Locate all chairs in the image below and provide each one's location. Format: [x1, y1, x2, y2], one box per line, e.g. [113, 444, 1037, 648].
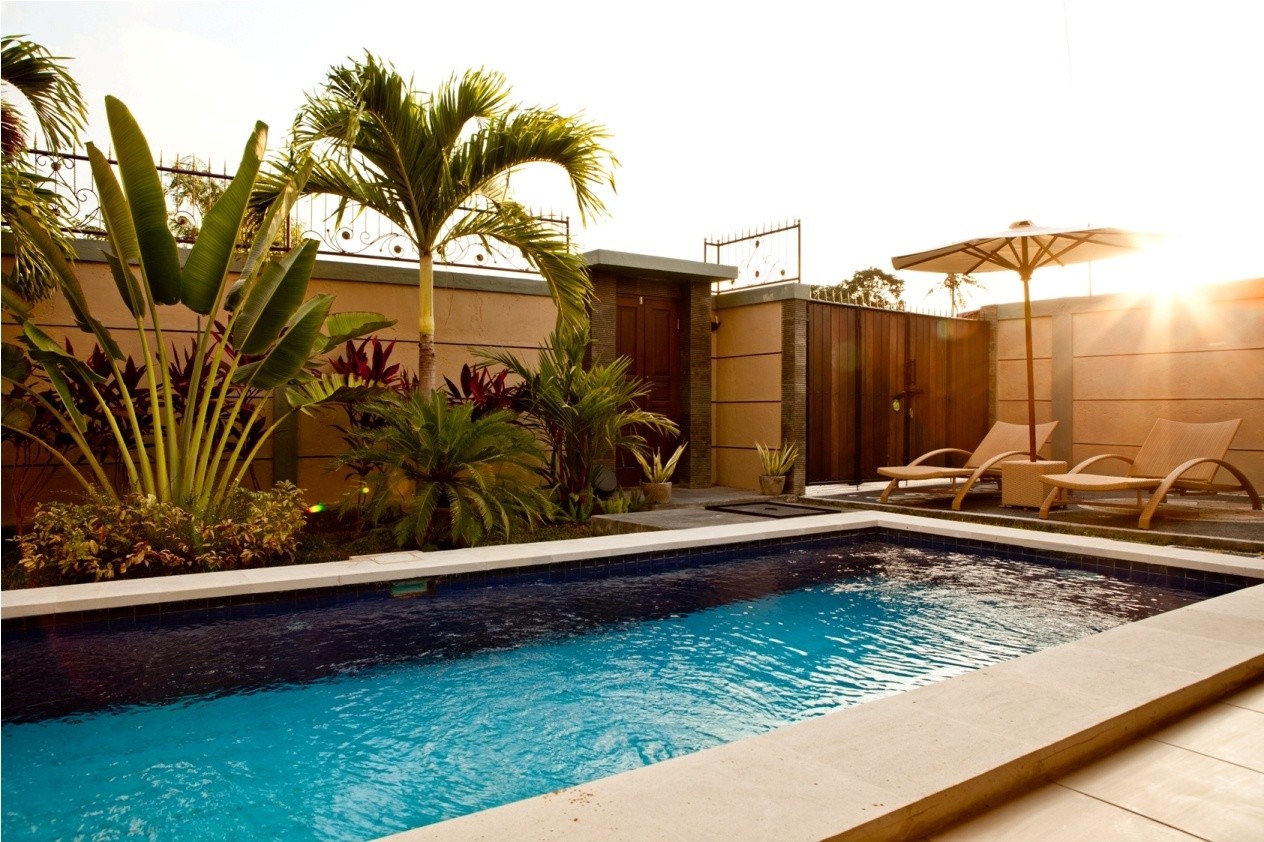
[1038, 418, 1263, 529]
[877, 420, 1059, 511]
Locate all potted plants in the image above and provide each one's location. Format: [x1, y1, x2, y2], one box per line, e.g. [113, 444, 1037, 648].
[629, 440, 689, 504]
[754, 438, 801, 495]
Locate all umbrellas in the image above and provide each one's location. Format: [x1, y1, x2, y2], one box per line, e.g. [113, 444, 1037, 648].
[891, 219, 1156, 462]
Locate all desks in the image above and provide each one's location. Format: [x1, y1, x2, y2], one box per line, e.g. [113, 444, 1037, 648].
[1001, 460, 1068, 510]
